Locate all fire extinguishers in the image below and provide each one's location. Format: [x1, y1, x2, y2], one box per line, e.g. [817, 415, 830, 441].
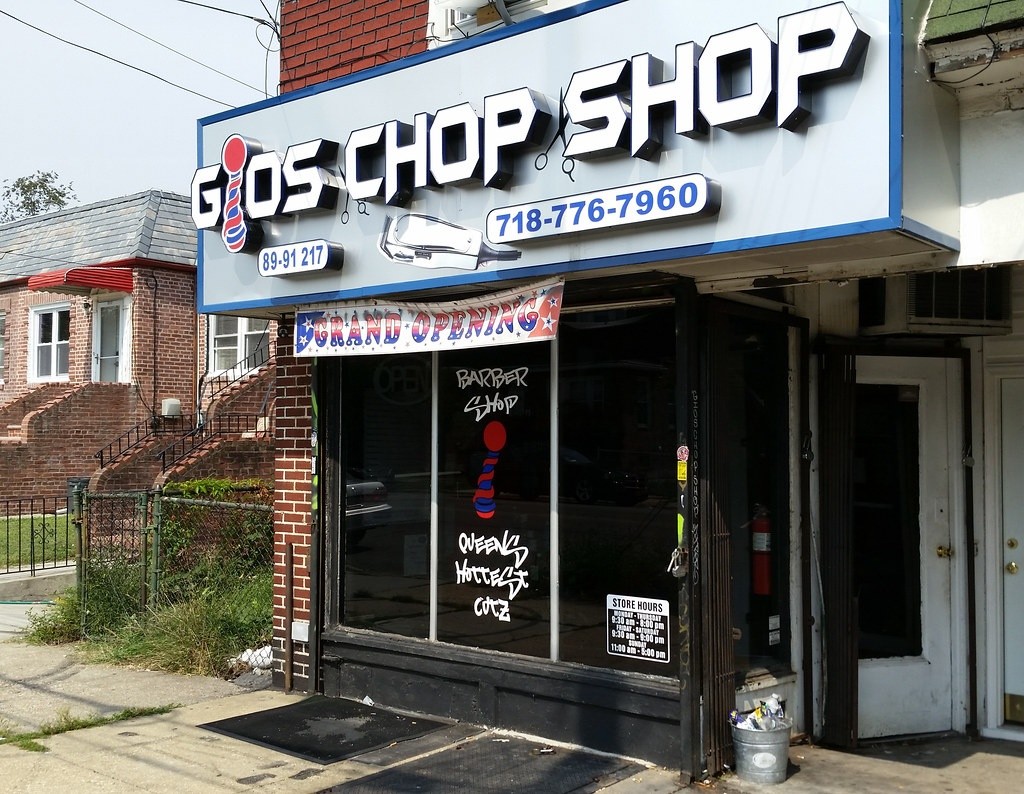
[750, 500, 775, 597]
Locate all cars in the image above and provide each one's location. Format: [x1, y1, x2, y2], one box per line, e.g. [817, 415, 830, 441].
[343, 470, 392, 551]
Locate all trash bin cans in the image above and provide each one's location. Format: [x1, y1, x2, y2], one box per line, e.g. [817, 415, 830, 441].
[728, 709, 795, 787]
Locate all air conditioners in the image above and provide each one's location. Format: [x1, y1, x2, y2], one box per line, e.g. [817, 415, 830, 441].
[859, 264, 1014, 337]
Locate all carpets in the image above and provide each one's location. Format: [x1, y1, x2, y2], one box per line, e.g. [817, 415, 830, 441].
[195, 695, 454, 766]
